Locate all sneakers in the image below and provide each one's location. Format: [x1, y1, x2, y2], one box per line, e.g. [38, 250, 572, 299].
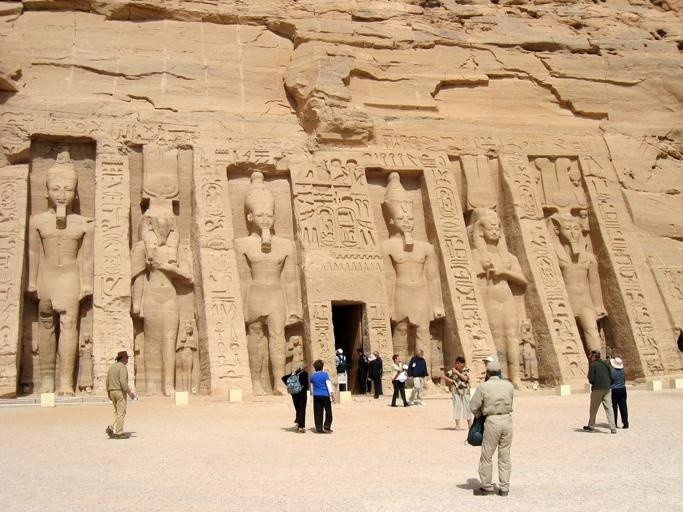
[111, 435, 127, 439]
[498, 489, 508, 496]
[105, 425, 113, 438]
[390, 402, 423, 407]
[473, 487, 494, 495]
[610, 424, 628, 434]
[294, 424, 333, 434]
[582, 426, 594, 432]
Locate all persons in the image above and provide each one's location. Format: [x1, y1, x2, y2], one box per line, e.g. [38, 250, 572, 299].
[609, 357, 628, 428]
[379, 171, 446, 373]
[548, 212, 609, 359]
[233, 170, 305, 396]
[440, 357, 472, 431]
[79, 332, 93, 392]
[368, 351, 384, 399]
[281, 364, 309, 433]
[407, 350, 429, 405]
[389, 354, 409, 407]
[104, 351, 135, 439]
[520, 319, 539, 380]
[176, 320, 196, 392]
[336, 348, 347, 373]
[482, 357, 504, 382]
[468, 361, 515, 497]
[24, 144, 93, 398]
[356, 349, 368, 395]
[466, 208, 527, 389]
[286, 336, 303, 373]
[130, 214, 193, 397]
[309, 359, 336, 434]
[583, 350, 617, 433]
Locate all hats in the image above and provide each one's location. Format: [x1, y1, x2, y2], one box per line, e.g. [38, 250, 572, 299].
[609, 358, 624, 370]
[115, 350, 130, 359]
[588, 351, 600, 359]
[482, 357, 495, 361]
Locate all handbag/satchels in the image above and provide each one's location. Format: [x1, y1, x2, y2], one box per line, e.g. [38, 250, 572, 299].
[466, 415, 486, 446]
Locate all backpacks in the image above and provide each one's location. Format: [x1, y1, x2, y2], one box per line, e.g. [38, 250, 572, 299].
[336, 355, 347, 371]
[286, 370, 304, 394]
